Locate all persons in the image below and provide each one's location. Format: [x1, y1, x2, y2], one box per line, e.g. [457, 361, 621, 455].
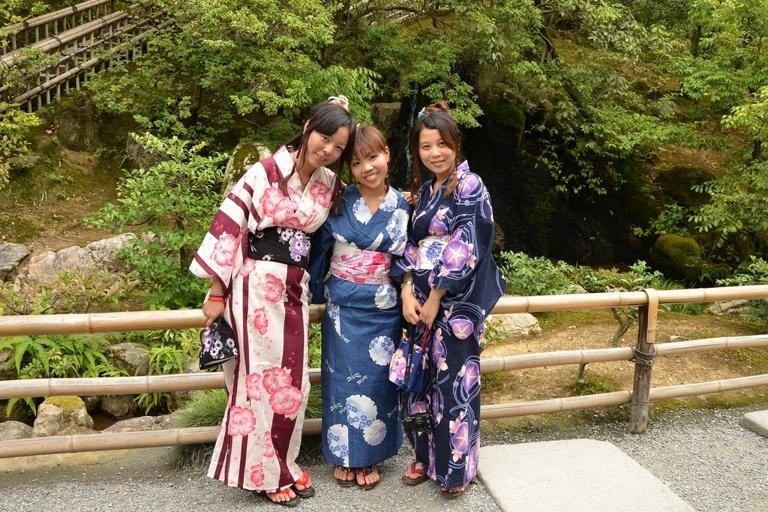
[389, 102, 507, 498]
[309, 124, 413, 490]
[189, 94, 419, 507]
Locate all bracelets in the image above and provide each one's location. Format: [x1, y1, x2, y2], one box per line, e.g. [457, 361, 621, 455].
[401, 279, 414, 285]
[207, 295, 225, 302]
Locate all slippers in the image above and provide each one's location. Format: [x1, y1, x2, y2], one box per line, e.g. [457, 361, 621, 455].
[402, 460, 430, 485]
[439, 479, 472, 498]
[265, 470, 315, 507]
[333, 465, 381, 491]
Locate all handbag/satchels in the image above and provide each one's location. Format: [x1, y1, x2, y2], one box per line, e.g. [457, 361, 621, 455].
[388, 320, 429, 396]
[199, 316, 239, 370]
